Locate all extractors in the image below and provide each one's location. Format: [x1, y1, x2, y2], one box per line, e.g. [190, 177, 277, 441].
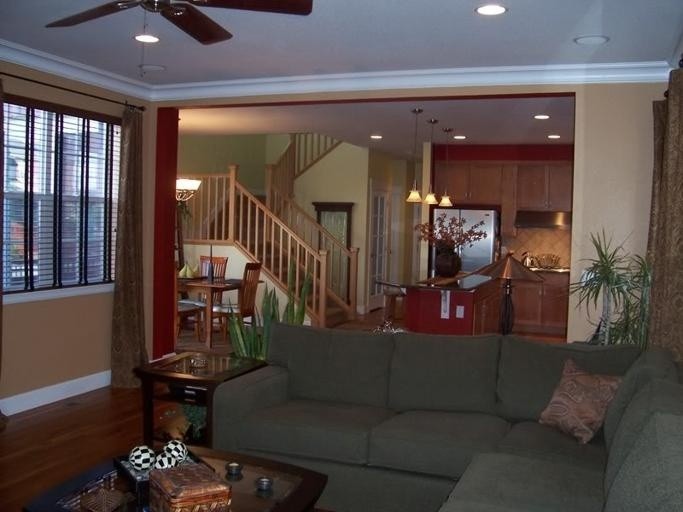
[514, 210, 572, 230]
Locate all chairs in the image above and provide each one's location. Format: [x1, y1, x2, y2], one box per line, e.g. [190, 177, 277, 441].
[175, 255, 263, 348]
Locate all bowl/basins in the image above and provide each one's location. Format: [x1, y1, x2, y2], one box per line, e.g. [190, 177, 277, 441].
[535, 254, 560, 269]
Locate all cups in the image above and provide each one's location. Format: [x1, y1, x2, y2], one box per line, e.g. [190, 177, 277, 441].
[255, 476, 273, 491]
[224, 461, 242, 475]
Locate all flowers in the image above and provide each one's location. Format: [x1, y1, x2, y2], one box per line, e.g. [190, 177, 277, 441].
[412, 213, 488, 253]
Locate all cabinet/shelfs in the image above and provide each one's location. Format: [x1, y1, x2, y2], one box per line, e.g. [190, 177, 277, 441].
[517, 164, 572, 208]
[435, 162, 501, 202]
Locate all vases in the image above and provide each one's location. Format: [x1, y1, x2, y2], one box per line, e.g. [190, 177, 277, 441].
[434, 247, 462, 278]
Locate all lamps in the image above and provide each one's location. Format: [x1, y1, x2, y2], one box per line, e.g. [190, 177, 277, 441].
[477, 252, 543, 336]
[405, 104, 453, 207]
[175, 178, 201, 202]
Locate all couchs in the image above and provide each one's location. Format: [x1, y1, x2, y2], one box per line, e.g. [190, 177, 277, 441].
[208, 320, 683, 511]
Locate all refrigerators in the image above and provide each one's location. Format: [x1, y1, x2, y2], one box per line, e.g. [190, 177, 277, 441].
[431, 209, 500, 275]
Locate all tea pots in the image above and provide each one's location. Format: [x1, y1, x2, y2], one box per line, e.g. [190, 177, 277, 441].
[521, 251, 535, 268]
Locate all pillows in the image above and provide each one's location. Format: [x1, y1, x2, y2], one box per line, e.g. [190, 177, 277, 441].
[539, 358, 624, 446]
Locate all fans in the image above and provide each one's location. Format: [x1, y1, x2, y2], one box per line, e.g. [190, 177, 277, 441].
[39, 0, 317, 45]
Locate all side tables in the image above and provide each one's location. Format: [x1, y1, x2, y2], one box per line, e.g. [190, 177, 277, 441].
[131, 349, 267, 451]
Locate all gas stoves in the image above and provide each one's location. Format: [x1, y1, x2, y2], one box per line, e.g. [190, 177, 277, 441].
[526, 267, 570, 273]
